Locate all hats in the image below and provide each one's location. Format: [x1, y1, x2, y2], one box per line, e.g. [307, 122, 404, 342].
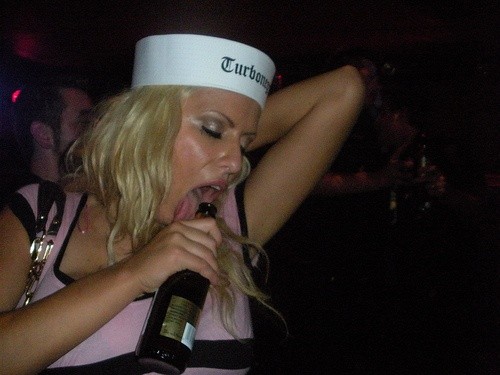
[133, 31, 275, 109]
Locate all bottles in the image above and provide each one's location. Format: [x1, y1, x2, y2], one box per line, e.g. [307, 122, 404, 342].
[415, 142, 429, 168]
[135, 202, 219, 374]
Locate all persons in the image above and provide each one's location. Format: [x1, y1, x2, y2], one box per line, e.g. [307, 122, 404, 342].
[1, 30, 368, 375]
[250, 43, 444, 375]
[0, 69, 94, 202]
